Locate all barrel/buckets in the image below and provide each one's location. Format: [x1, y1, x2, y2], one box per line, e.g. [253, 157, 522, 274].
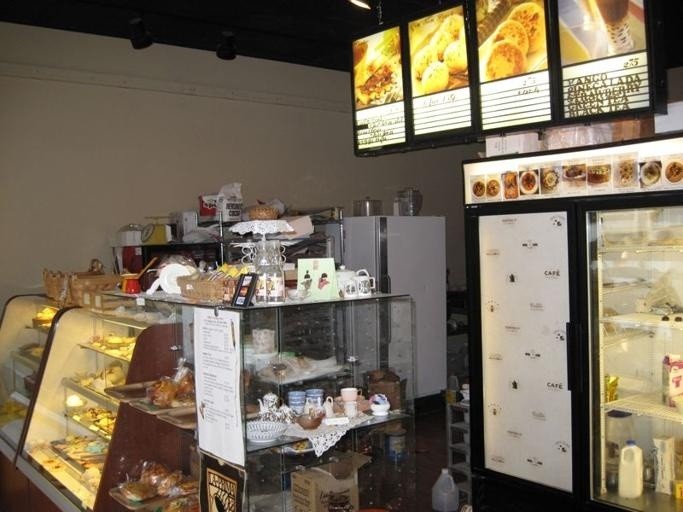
[618, 440, 644, 497]
[432, 468, 458, 511]
[398, 190, 421, 216]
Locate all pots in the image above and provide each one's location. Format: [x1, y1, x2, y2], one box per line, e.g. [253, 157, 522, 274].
[352, 196, 383, 216]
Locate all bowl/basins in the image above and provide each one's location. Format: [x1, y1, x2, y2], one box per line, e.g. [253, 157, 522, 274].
[485, 180, 499, 198]
[639, 162, 660, 186]
[663, 160, 682, 183]
[286, 389, 324, 415]
[471, 182, 485, 199]
[245, 419, 288, 444]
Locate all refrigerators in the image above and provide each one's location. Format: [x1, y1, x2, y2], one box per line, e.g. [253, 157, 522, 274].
[460, 129, 679, 512]
[324, 216, 447, 416]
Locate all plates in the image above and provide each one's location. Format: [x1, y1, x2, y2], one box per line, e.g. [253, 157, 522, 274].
[561, 171, 586, 180]
[333, 395, 365, 405]
[519, 170, 539, 196]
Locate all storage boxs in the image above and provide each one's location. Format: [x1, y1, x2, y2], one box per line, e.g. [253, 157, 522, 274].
[363, 369, 407, 412]
[289, 452, 370, 511]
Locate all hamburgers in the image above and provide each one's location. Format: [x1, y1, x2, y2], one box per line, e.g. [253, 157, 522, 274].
[354, 52, 397, 105]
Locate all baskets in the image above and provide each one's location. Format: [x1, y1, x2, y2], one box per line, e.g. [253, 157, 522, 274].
[70, 271, 126, 307]
[176, 275, 233, 303]
[41, 267, 76, 307]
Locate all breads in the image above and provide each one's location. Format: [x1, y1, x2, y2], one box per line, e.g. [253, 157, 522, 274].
[148, 372, 195, 407]
[413, 14, 468, 94]
[119, 464, 200, 512]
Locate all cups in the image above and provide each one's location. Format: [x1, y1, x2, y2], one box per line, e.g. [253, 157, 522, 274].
[340, 387, 363, 400]
[370, 403, 390, 416]
[296, 413, 321, 430]
[336, 265, 377, 298]
[119, 273, 141, 293]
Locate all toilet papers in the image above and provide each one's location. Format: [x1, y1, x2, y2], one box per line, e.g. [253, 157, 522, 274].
[146, 264, 189, 296]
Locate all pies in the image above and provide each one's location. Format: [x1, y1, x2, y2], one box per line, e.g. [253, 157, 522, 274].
[486, 2, 546, 81]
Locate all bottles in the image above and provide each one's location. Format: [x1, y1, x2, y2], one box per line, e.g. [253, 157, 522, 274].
[253, 255, 285, 302]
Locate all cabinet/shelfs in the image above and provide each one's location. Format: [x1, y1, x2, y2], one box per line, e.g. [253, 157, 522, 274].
[444, 402, 471, 505]
[191, 289, 417, 512]
[1, 292, 68, 511]
[7, 307, 192, 511]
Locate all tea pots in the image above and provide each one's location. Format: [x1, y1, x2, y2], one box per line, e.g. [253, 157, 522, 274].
[256, 392, 283, 418]
